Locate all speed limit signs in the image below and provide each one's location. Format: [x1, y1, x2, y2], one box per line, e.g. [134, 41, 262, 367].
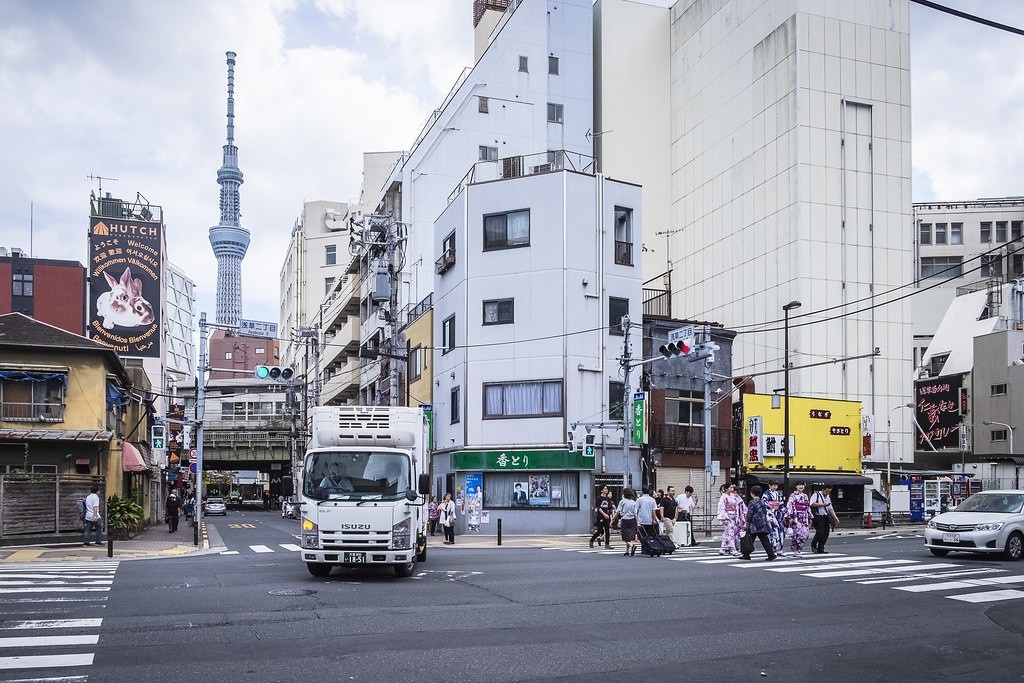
[189, 448, 197, 459]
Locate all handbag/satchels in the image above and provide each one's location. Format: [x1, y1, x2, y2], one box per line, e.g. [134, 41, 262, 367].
[828, 513, 834, 523]
[738, 530, 754, 553]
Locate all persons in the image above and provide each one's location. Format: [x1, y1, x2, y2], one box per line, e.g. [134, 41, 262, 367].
[83, 486, 106, 547]
[676, 485, 700, 546]
[615, 485, 664, 557]
[438, 493, 456, 544]
[597, 491, 617, 546]
[165, 489, 182, 533]
[429, 496, 440, 536]
[717, 482, 839, 562]
[183, 493, 194, 515]
[319, 462, 354, 491]
[589, 485, 615, 549]
[238, 493, 244, 501]
[530, 476, 549, 497]
[475, 485, 481, 498]
[370, 462, 401, 492]
[513, 483, 526, 501]
[263, 492, 284, 510]
[660, 486, 679, 549]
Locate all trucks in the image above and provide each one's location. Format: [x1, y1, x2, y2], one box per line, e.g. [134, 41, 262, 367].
[282, 405, 431, 577]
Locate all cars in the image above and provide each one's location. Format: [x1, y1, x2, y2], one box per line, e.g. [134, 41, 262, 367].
[227, 492, 240, 503]
[203, 498, 227, 516]
[923, 490, 1024, 561]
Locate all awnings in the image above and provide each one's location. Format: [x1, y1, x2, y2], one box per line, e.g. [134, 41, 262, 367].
[122, 442, 153, 475]
[748, 474, 873, 485]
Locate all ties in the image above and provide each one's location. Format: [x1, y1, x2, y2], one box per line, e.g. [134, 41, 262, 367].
[445, 504, 448, 520]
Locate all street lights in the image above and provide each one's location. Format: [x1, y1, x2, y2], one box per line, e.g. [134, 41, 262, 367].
[887, 402, 917, 493]
[983, 420, 1013, 454]
[783, 300, 802, 508]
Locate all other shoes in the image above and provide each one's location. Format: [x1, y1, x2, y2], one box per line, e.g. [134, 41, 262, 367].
[778, 552, 787, 557]
[719, 550, 724, 555]
[589, 540, 594, 548]
[444, 542, 454, 544]
[631, 546, 637, 556]
[693, 542, 699, 546]
[83, 543, 93, 547]
[605, 544, 614, 549]
[818, 550, 828, 554]
[811, 546, 818, 554]
[765, 555, 776, 561]
[739, 555, 750, 560]
[624, 553, 629, 556]
[96, 542, 105, 547]
[733, 552, 739, 556]
[794, 551, 801, 556]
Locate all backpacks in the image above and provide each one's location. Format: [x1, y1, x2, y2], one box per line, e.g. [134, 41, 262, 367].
[78, 498, 87, 519]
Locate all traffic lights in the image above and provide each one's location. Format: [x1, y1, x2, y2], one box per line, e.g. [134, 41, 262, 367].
[582, 434, 596, 457]
[567, 431, 578, 453]
[255, 365, 295, 382]
[659, 338, 693, 360]
[151, 425, 166, 451]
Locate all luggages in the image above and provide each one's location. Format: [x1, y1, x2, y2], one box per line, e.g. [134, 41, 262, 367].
[637, 525, 663, 557]
[675, 519, 692, 547]
[652, 524, 676, 554]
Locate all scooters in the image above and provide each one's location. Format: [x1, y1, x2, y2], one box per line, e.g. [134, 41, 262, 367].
[281, 500, 295, 519]
